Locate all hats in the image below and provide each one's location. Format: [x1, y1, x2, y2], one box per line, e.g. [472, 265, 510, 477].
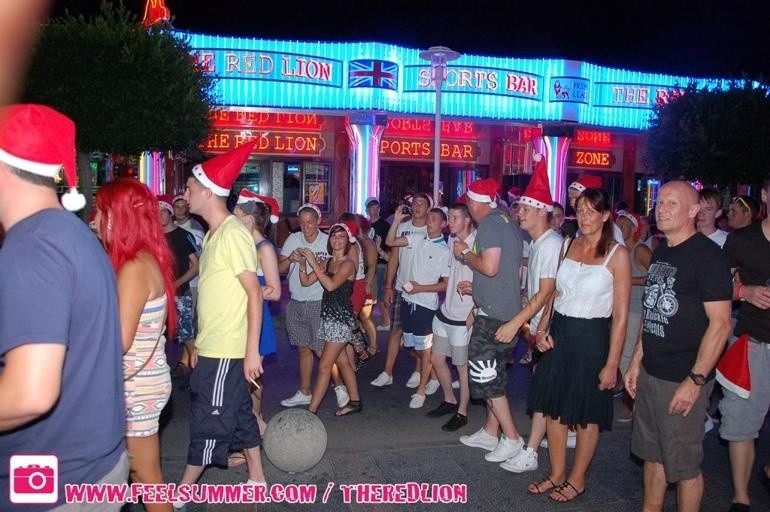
[172, 192, 185, 205]
[508, 185, 522, 198]
[157, 193, 175, 217]
[236, 188, 280, 225]
[519, 152, 554, 213]
[1, 102, 87, 212]
[297, 201, 322, 219]
[328, 220, 357, 243]
[616, 209, 643, 240]
[467, 177, 500, 208]
[568, 174, 602, 193]
[192, 136, 260, 198]
[715, 332, 752, 400]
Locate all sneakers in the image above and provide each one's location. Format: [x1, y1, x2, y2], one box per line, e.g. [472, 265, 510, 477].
[408, 394, 426, 409]
[459, 426, 499, 452]
[424, 379, 441, 396]
[425, 401, 459, 418]
[405, 371, 421, 389]
[485, 432, 526, 463]
[281, 389, 313, 408]
[441, 413, 468, 432]
[370, 371, 394, 387]
[617, 407, 633, 423]
[376, 324, 391, 332]
[539, 428, 577, 449]
[498, 444, 539, 474]
[333, 385, 350, 408]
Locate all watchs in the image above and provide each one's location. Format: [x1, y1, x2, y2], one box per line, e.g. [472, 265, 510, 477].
[688, 370, 706, 386]
[460, 249, 472, 263]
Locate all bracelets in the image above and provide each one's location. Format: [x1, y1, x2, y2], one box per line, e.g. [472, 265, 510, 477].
[300, 270, 307, 273]
[536, 330, 546, 333]
[316, 270, 323, 277]
[736, 284, 744, 300]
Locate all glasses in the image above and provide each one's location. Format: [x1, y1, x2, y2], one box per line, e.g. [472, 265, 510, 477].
[576, 208, 596, 216]
[733, 196, 751, 212]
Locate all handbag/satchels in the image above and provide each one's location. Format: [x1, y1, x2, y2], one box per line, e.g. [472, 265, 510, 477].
[325, 257, 367, 354]
[530, 235, 576, 364]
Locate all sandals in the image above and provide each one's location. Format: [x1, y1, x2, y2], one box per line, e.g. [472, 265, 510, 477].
[366, 345, 381, 356]
[334, 398, 364, 417]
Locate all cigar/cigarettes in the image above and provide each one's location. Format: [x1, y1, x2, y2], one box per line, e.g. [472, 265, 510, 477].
[249, 377, 261, 388]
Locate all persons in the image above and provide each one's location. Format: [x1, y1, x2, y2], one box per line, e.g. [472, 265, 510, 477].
[366, 197, 393, 331]
[724, 195, 759, 228]
[385, 202, 441, 410]
[428, 203, 478, 430]
[354, 212, 381, 358]
[173, 140, 267, 507]
[494, 154, 565, 473]
[233, 189, 283, 436]
[297, 218, 363, 417]
[623, 180, 733, 512]
[93, 180, 178, 511]
[527, 185, 632, 502]
[453, 178, 525, 462]
[338, 220, 367, 372]
[618, 214, 652, 422]
[570, 176, 626, 248]
[154, 195, 199, 374]
[371, 195, 434, 387]
[696, 189, 729, 246]
[720, 172, 770, 512]
[1, 105, 131, 512]
[173, 198, 205, 313]
[278, 202, 350, 409]
[551, 201, 564, 233]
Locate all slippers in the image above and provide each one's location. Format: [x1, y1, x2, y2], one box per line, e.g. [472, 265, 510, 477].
[549, 480, 586, 502]
[225, 450, 247, 467]
[528, 475, 561, 494]
[519, 352, 533, 365]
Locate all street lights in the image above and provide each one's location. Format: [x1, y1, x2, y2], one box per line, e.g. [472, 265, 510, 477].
[417, 44, 461, 207]
